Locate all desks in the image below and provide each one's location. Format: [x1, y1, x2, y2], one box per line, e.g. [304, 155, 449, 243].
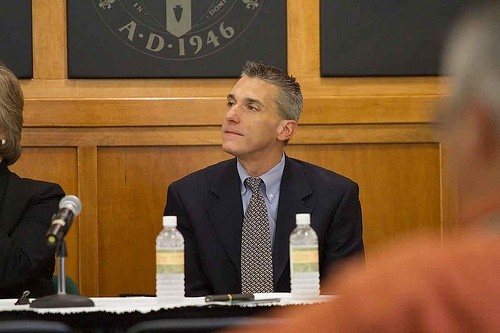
[0, 293, 339, 333]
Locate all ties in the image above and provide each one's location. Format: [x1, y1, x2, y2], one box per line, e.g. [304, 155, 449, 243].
[241, 177, 274, 296]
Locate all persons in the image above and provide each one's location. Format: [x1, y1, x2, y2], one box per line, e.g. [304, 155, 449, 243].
[231, 9, 500, 333]
[163, 60, 372, 297]
[0, 66, 67, 298]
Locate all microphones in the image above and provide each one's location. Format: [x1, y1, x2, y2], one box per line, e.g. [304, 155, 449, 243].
[46, 194, 82, 246]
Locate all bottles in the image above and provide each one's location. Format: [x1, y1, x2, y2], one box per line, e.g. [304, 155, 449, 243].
[156, 216, 186, 305]
[289, 214, 320, 297]
